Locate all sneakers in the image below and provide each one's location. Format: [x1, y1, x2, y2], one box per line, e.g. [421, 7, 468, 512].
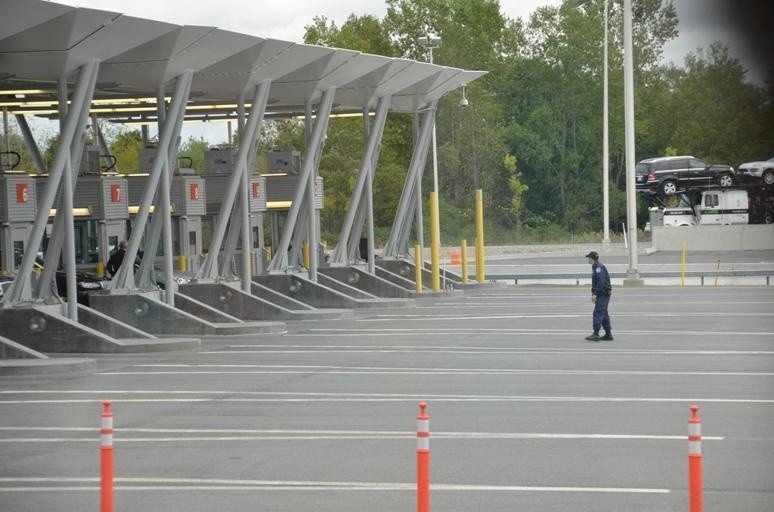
[586, 334, 600, 340]
[600, 335, 613, 340]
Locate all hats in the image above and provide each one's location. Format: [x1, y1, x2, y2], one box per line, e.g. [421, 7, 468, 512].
[585, 252, 598, 259]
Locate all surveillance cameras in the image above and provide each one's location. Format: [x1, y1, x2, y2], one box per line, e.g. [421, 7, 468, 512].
[460, 97, 468, 108]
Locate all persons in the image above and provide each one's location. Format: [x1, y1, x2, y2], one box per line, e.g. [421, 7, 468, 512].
[585, 250, 614, 340]
[105, 239, 142, 276]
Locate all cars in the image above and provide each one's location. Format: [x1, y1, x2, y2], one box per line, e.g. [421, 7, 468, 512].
[746, 193, 774, 224]
[739, 158, 774, 188]
[635, 154, 738, 199]
